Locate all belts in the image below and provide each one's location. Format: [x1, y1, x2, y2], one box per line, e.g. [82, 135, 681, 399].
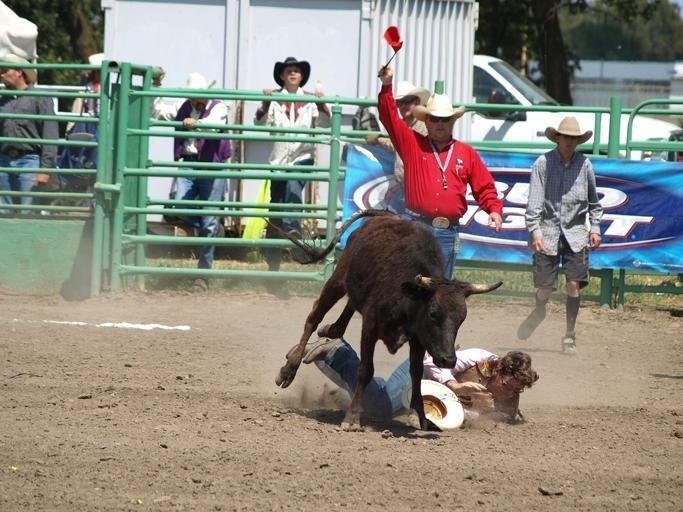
[404, 211, 467, 230]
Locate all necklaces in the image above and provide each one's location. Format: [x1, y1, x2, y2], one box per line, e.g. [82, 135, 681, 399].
[515, 115, 602, 358]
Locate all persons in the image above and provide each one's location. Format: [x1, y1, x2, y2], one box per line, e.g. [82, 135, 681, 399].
[251, 55, 333, 272]
[58, 52, 113, 196]
[374, 64, 504, 283]
[147, 65, 232, 294]
[0, 52, 59, 216]
[280, 334, 540, 432]
[362, 81, 432, 186]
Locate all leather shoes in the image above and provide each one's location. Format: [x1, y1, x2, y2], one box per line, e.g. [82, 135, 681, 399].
[301, 336, 345, 364]
[284, 336, 336, 360]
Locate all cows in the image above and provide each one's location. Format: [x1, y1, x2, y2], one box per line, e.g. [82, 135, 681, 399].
[262, 208, 504, 433]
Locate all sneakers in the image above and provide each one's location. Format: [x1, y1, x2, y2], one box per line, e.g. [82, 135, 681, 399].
[560, 335, 578, 356]
[517, 307, 547, 341]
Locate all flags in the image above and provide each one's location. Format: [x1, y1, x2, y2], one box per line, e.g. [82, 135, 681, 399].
[383, 24, 402, 52]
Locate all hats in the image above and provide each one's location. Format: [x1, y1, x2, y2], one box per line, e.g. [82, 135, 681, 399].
[394, 82, 469, 124]
[2, 52, 35, 82]
[180, 70, 218, 92]
[544, 115, 594, 146]
[405, 377, 465, 430]
[80, 52, 104, 75]
[274, 58, 309, 86]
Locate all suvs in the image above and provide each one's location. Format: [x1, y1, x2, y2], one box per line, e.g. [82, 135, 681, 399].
[470, 48, 683, 162]
